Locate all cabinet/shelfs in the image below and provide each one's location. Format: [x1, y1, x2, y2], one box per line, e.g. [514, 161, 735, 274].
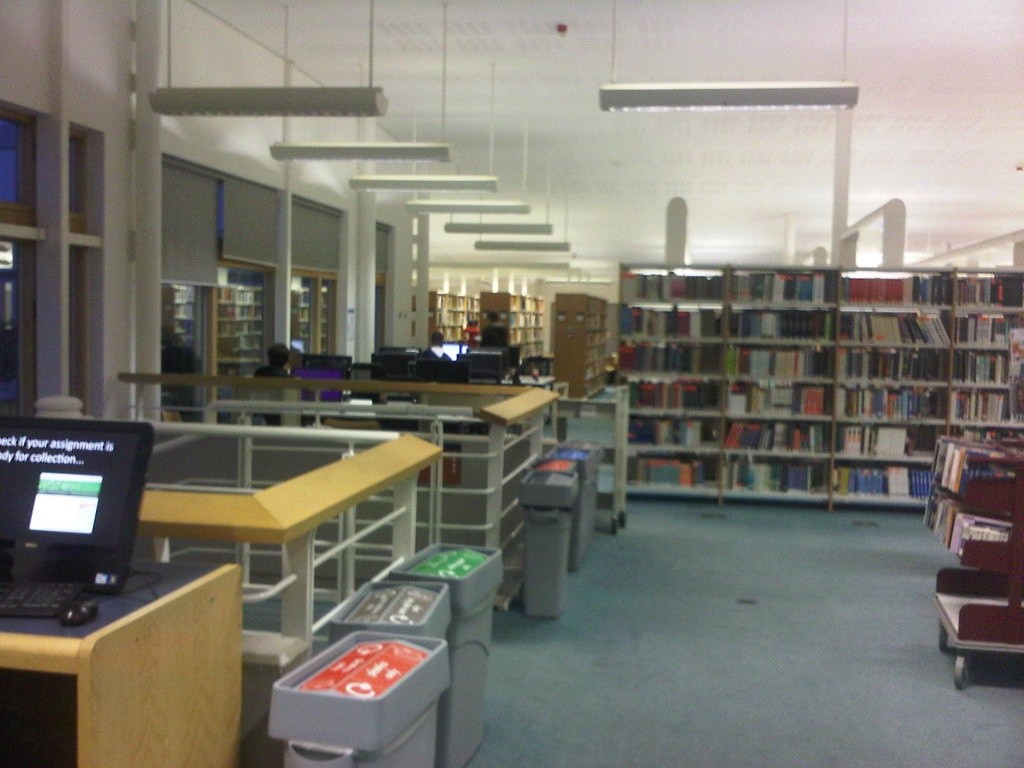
[199, 250, 385, 401]
[410, 257, 1023, 692]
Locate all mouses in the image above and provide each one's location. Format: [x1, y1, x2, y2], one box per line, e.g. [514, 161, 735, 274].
[58, 596, 99, 627]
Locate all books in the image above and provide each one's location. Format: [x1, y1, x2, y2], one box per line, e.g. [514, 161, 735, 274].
[433, 294, 608, 398]
[170, 284, 331, 426]
[620, 271, 1024, 556]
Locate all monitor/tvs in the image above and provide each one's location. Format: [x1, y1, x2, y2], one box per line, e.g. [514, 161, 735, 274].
[0, 411, 153, 586]
[302, 340, 520, 385]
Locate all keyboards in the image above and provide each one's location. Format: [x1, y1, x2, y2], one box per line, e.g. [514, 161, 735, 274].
[0, 579, 91, 617]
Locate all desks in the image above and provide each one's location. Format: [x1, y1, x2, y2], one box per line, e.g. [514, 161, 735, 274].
[1, 557, 244, 768]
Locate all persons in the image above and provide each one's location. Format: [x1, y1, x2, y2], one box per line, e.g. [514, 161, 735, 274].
[481, 311, 507, 349]
[254, 344, 299, 425]
[461, 320, 480, 348]
[421, 332, 451, 361]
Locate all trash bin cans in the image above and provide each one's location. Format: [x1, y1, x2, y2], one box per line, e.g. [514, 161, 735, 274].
[269, 581, 453, 768]
[521, 438, 603, 617]
[390, 542, 503, 768]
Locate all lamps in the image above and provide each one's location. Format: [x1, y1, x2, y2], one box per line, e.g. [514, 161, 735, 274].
[150, 0, 573, 253]
[599, 0, 860, 115]
